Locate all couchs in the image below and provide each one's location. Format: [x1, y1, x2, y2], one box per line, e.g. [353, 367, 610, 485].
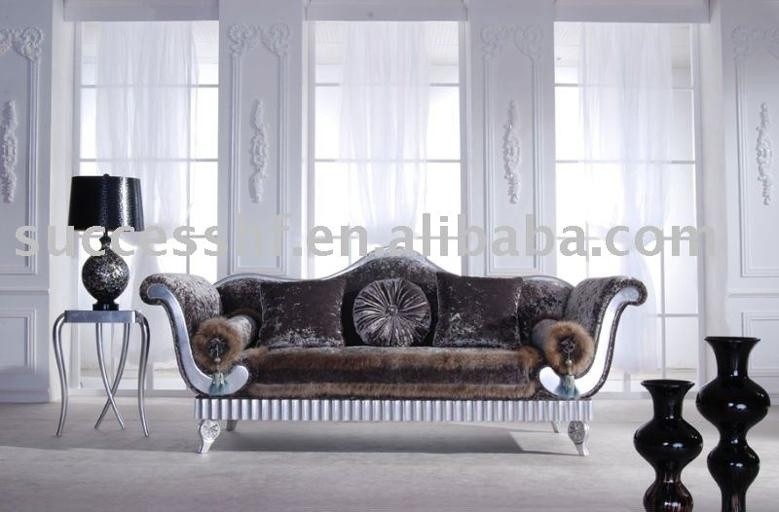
[138, 242, 648, 457]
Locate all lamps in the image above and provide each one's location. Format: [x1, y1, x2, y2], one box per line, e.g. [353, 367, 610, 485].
[67, 174, 144, 310]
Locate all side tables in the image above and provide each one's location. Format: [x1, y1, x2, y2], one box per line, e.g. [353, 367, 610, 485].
[53, 310, 150, 439]
